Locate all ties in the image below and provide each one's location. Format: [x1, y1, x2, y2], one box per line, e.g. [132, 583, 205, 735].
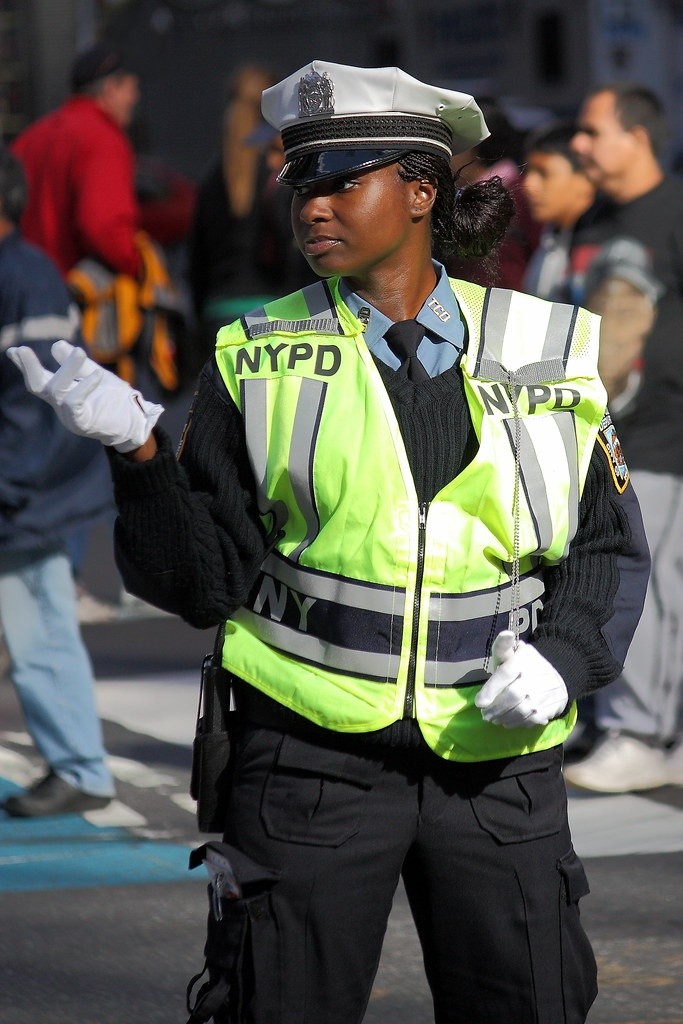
[383, 319, 431, 385]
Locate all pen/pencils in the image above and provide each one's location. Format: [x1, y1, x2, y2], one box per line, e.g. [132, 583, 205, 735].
[212, 872, 228, 924]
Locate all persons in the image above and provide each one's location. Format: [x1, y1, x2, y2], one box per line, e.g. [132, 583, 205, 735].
[1, 38, 682, 1024]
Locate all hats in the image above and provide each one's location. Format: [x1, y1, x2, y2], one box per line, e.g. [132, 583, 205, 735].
[260, 60, 493, 187]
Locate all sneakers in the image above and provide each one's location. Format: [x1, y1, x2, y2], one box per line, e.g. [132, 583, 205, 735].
[562, 733, 683, 795]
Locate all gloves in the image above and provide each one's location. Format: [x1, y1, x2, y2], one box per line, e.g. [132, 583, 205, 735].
[6, 338, 166, 454]
[472, 629, 569, 731]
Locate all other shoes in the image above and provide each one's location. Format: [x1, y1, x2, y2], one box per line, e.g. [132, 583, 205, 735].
[4, 769, 113, 816]
